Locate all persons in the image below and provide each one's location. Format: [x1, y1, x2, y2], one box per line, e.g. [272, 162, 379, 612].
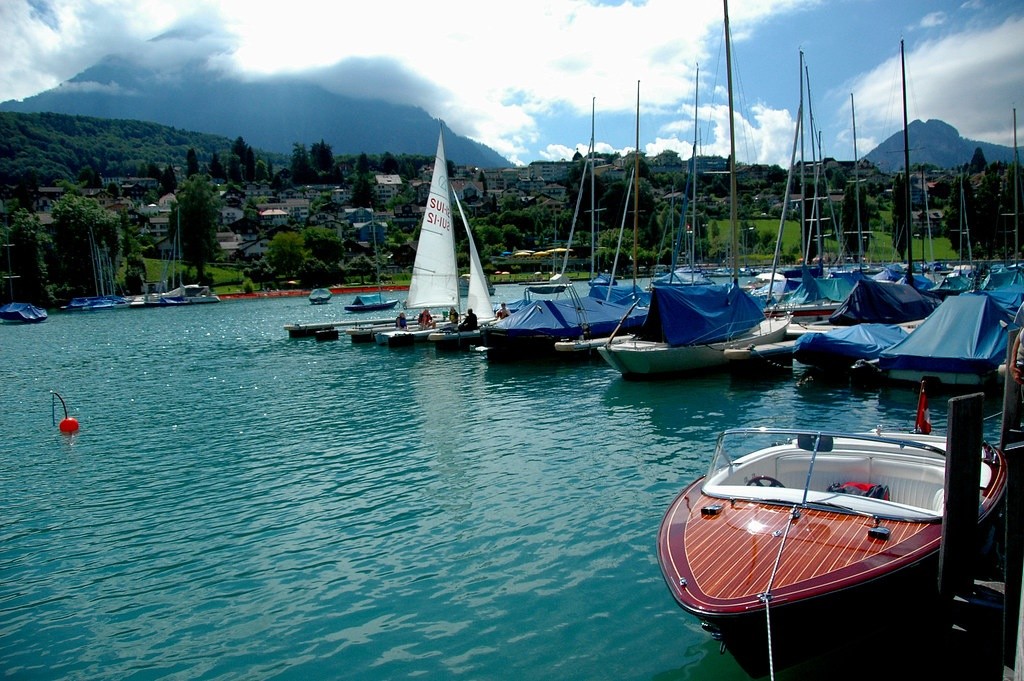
[419, 309, 436, 328]
[395, 312, 406, 328]
[496, 303, 511, 319]
[1009, 328, 1024, 430]
[449, 307, 458, 324]
[458, 308, 477, 331]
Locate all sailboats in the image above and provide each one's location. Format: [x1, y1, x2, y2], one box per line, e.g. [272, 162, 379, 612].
[64, 204, 221, 311]
[343, 204, 399, 311]
[374, 0, 1024, 393]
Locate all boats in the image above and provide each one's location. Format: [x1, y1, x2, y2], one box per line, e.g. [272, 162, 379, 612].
[0, 302, 48, 325]
[309, 288, 333, 305]
[656, 378, 1010, 639]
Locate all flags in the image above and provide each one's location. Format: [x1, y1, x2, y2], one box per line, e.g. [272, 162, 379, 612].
[918, 388, 931, 434]
